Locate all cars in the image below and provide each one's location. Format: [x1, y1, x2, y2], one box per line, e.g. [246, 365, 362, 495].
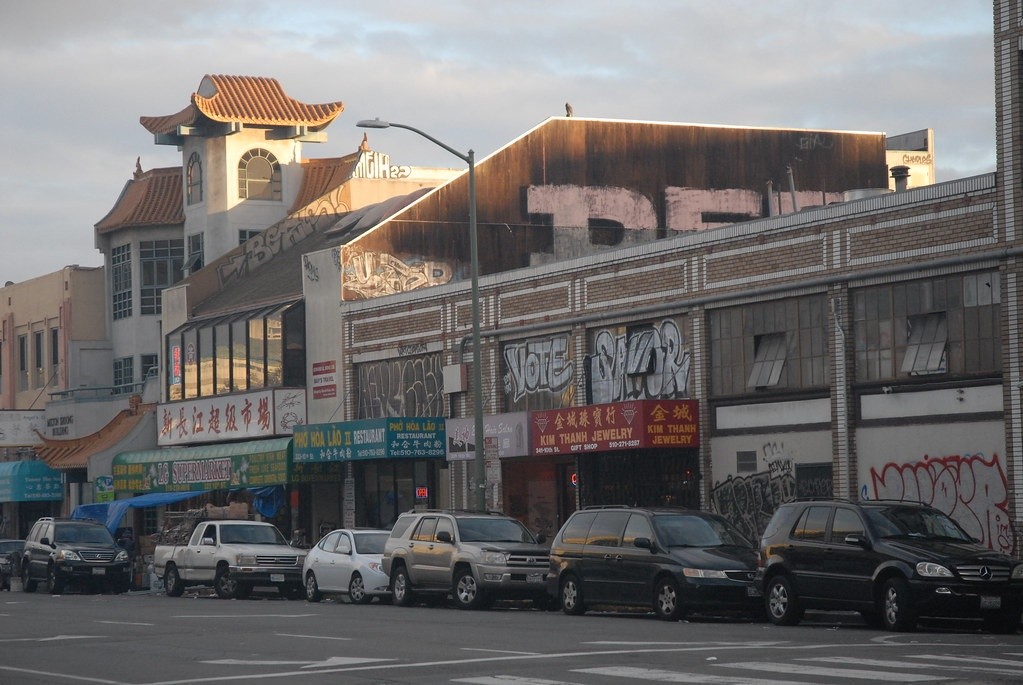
[0, 539, 29, 585]
[300, 528, 396, 602]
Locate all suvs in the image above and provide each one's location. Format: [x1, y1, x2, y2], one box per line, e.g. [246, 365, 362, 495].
[551, 502, 762, 624]
[21, 516, 133, 595]
[756, 496, 1023, 637]
[382, 510, 556, 608]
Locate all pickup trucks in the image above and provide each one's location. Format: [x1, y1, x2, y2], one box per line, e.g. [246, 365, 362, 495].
[153, 519, 309, 601]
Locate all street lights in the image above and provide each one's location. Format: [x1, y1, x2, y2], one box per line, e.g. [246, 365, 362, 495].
[355, 118, 492, 510]
